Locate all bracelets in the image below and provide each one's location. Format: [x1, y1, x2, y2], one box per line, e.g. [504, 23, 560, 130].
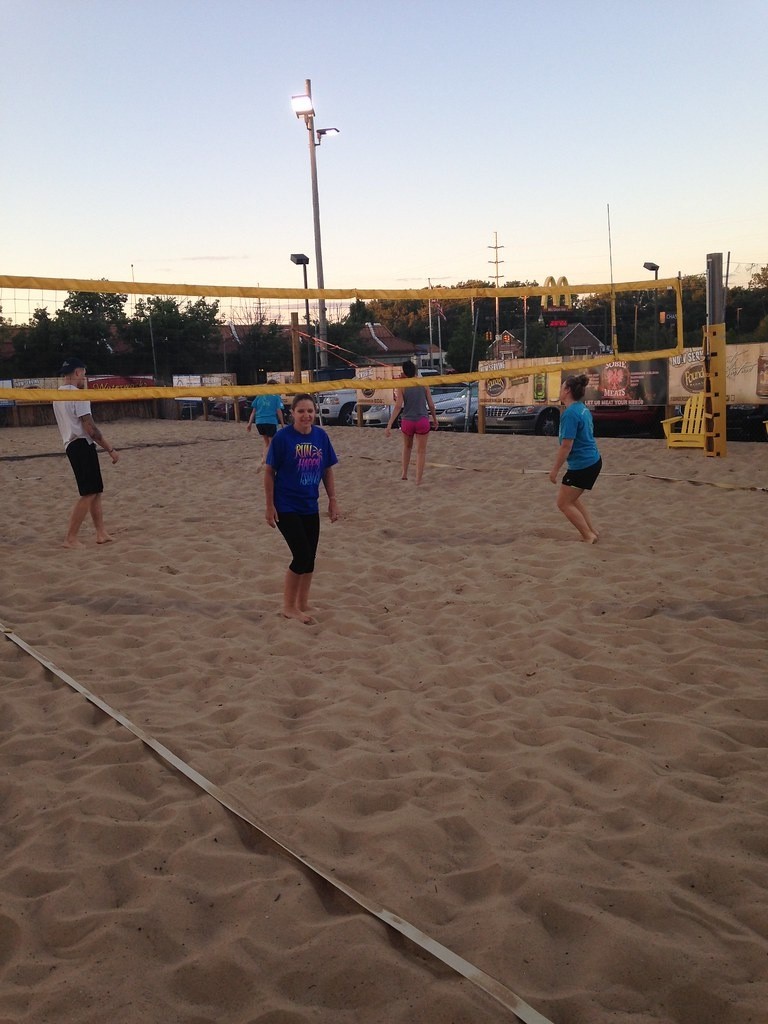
[330, 497, 335, 499]
[111, 449, 113, 452]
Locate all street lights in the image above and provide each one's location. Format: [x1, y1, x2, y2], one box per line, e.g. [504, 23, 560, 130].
[290, 79, 339, 367]
[291, 253, 313, 370]
[643, 262, 660, 365]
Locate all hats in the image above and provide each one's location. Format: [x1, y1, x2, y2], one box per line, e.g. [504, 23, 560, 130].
[62, 358, 85, 374]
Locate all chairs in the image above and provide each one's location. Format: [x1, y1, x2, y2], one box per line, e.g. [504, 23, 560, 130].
[660, 391, 706, 451]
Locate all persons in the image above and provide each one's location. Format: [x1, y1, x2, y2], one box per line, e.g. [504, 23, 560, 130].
[53, 357, 119, 549]
[549, 375, 602, 544]
[245, 379, 285, 464]
[264, 394, 339, 624]
[385, 361, 437, 485]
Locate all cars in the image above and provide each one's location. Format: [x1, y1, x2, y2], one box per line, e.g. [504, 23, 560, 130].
[590, 406, 659, 437]
[211, 397, 247, 421]
[314, 389, 358, 426]
[351, 382, 470, 429]
[474, 406, 560, 436]
[674, 404, 768, 442]
[426, 381, 479, 431]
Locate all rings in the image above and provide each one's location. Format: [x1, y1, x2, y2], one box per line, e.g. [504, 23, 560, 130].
[266, 520, 268, 521]
[336, 514, 339, 515]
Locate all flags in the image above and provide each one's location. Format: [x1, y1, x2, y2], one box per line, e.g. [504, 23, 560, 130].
[431, 285, 447, 320]
